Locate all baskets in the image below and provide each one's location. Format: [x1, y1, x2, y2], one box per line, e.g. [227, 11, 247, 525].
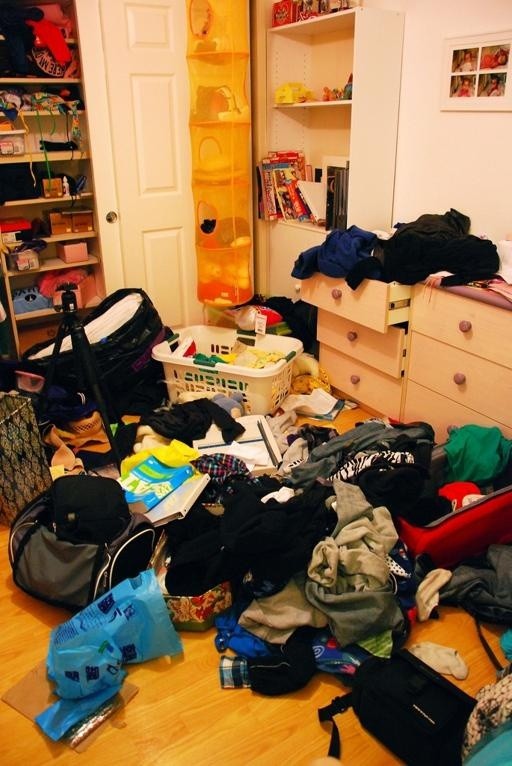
[150, 324, 305, 417]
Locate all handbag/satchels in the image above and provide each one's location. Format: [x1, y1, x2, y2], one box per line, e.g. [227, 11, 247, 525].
[352, 649, 477, 765]
[8, 475, 158, 614]
[17, 287, 179, 426]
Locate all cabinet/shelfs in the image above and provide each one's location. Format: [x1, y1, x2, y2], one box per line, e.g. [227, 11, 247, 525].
[265, 6, 407, 304]
[0, 0, 110, 361]
[297, 271, 512, 448]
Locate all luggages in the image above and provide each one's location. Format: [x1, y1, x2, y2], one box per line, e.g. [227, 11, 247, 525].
[379, 437, 512, 569]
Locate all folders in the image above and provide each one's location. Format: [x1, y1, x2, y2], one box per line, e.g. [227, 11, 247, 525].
[144, 473, 211, 528]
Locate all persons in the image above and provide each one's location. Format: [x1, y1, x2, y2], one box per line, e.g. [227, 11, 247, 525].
[449, 42, 509, 97]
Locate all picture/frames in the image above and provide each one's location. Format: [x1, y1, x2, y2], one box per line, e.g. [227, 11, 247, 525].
[438, 30, 512, 112]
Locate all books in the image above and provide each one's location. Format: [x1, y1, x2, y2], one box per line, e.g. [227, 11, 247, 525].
[254, 149, 348, 233]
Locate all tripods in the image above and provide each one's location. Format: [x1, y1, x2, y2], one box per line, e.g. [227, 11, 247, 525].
[41, 282, 131, 476]
